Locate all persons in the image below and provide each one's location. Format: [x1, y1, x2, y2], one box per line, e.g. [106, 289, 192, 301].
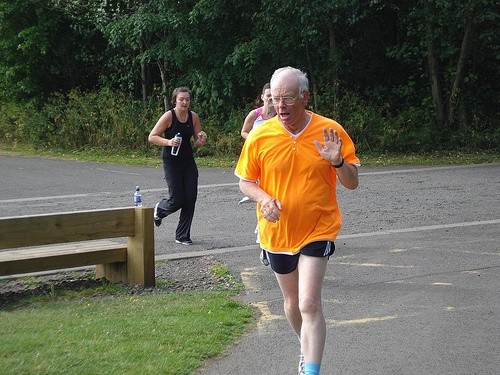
[233, 66, 361, 375]
[148, 87, 207, 246]
[237, 83, 278, 205]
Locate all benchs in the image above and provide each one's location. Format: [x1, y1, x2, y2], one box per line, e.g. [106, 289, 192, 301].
[0, 205, 154, 286]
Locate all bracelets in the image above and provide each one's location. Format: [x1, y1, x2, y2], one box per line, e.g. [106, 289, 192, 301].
[332, 158, 344, 168]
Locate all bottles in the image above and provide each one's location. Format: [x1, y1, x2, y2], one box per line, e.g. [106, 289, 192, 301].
[170, 133, 182, 156]
[133, 186, 142, 207]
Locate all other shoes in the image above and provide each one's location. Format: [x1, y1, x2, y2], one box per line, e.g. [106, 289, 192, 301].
[154, 202, 162, 227]
[175, 239, 193, 245]
[298, 353, 305, 375]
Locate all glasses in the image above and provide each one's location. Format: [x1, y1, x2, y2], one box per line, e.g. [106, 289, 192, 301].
[268, 96, 299, 107]
